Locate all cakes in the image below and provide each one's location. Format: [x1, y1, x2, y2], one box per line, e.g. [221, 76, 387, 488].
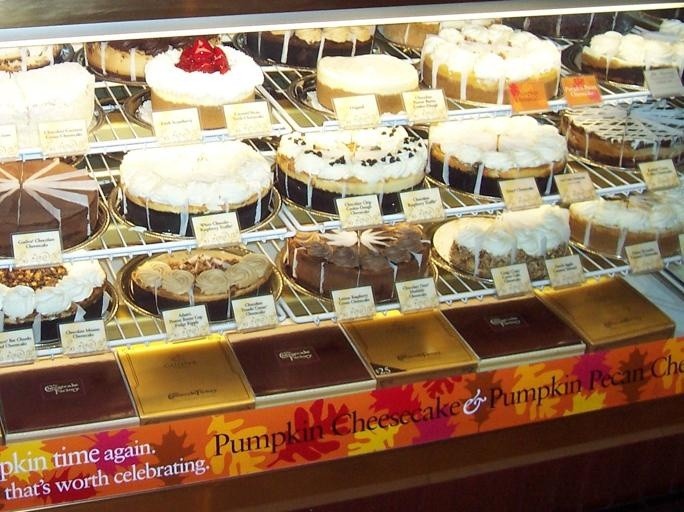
[560, 98, 683, 167]
[450, 205, 572, 279]
[317, 55, 419, 114]
[377, 18, 499, 47]
[144, 47, 264, 130]
[129, 249, 272, 321]
[1, 62, 96, 155]
[1, 260, 107, 339]
[569, 186, 683, 258]
[276, 125, 428, 216]
[234, 24, 376, 68]
[582, 18, 683, 84]
[118, 141, 274, 237]
[421, 23, 562, 107]
[1, 43, 74, 71]
[429, 116, 569, 198]
[284, 221, 431, 306]
[1, 159, 98, 258]
[84, 34, 219, 87]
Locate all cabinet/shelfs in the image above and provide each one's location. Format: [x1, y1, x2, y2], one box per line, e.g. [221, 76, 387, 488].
[1, 2, 682, 511]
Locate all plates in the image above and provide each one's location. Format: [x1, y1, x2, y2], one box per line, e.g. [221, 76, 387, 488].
[75, 47, 148, 86]
[0, 198, 110, 260]
[86, 100, 105, 137]
[562, 148, 681, 171]
[33, 279, 117, 347]
[428, 215, 573, 284]
[427, 163, 575, 199]
[117, 246, 283, 320]
[289, 72, 334, 116]
[276, 246, 437, 304]
[281, 191, 341, 222]
[122, 88, 155, 133]
[561, 40, 645, 90]
[109, 184, 284, 240]
